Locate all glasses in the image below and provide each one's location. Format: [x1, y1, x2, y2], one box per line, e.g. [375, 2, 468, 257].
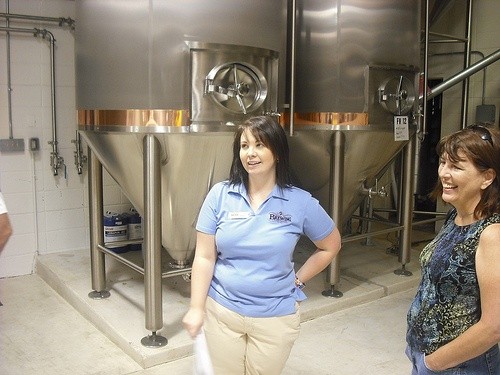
[467, 124, 494, 148]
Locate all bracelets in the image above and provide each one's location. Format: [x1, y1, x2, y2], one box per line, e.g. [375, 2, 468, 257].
[295, 277, 306, 290]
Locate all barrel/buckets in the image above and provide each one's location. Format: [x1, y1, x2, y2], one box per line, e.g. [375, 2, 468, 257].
[104, 211, 127, 253]
[121, 208, 147, 251]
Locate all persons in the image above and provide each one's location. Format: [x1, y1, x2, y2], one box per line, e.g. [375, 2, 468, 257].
[0, 196, 13, 255]
[405, 124, 500, 375]
[182, 115, 342, 375]
